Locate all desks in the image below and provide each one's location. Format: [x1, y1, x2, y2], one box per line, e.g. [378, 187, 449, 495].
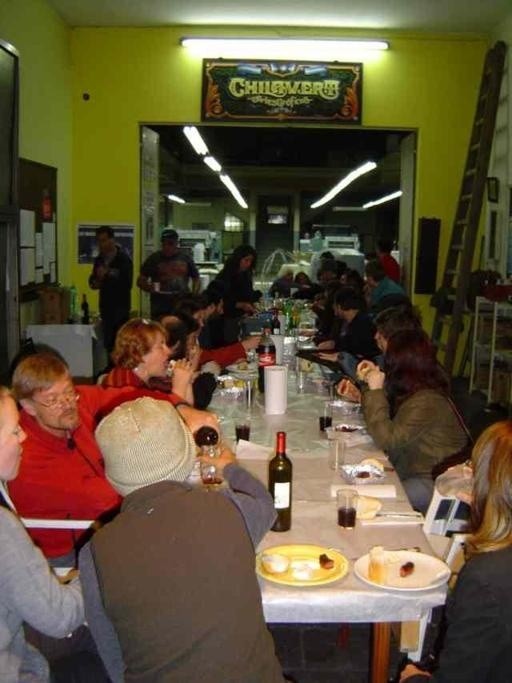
[26, 317, 103, 378]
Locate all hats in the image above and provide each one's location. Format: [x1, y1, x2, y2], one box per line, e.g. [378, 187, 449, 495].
[95, 397, 196, 497]
[161, 230, 178, 241]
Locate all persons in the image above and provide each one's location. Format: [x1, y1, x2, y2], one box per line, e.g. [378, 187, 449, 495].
[4, 350, 222, 565]
[0, 381, 87, 682]
[267, 251, 469, 510]
[88, 226, 263, 409]
[76, 396, 290, 682]
[397, 420, 512, 683]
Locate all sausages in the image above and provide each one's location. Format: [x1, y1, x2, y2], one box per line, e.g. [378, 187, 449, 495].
[399, 562, 416, 577]
[319, 554, 335, 569]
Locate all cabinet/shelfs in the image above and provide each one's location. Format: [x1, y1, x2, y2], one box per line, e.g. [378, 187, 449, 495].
[468, 295, 512, 411]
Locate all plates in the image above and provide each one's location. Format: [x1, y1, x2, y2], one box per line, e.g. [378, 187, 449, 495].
[324, 399, 362, 415]
[352, 549, 452, 593]
[325, 422, 366, 439]
[253, 542, 350, 590]
[224, 363, 257, 373]
[337, 461, 389, 486]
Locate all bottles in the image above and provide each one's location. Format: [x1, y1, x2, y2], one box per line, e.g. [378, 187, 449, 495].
[194, 425, 219, 447]
[268, 431, 293, 532]
[81, 293, 90, 324]
[258, 328, 277, 394]
[269, 328, 283, 365]
[281, 297, 307, 333]
[262, 297, 281, 327]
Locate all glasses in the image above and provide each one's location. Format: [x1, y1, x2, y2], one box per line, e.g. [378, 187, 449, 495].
[30, 386, 80, 414]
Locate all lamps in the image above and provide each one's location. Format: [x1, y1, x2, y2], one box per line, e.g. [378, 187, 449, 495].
[176, 32, 392, 54]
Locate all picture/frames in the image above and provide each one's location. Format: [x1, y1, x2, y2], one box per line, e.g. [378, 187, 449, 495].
[486, 177, 499, 203]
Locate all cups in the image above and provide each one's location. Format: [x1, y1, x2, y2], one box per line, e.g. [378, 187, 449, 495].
[241, 380, 257, 408]
[336, 489, 358, 528]
[199, 445, 228, 484]
[328, 438, 346, 473]
[235, 420, 251, 443]
[294, 370, 308, 394]
[318, 402, 332, 433]
[264, 366, 289, 415]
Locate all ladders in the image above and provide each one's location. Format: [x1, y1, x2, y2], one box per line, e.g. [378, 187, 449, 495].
[431, 38, 512, 386]
